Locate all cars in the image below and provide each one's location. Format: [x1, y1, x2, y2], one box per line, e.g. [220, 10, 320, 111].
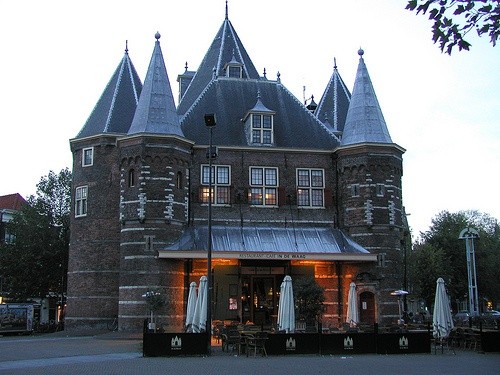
[481, 310, 500, 321]
[410, 310, 434, 324]
[454, 309, 470, 321]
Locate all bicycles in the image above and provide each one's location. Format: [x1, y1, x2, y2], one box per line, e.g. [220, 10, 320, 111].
[32, 321, 63, 336]
[106, 314, 119, 331]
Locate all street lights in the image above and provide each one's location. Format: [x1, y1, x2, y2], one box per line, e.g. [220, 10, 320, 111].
[203, 113, 219, 355]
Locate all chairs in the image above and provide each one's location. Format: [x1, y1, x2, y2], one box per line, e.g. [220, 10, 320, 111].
[306, 321, 359, 333]
[434, 328, 481, 356]
[213, 321, 269, 360]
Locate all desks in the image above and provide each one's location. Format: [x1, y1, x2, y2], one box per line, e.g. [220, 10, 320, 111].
[239, 331, 257, 358]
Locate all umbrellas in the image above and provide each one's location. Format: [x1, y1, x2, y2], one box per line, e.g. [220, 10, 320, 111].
[433, 278, 453, 355]
[277, 276, 295, 333]
[191, 276, 207, 333]
[346, 282, 359, 327]
[186, 282, 196, 333]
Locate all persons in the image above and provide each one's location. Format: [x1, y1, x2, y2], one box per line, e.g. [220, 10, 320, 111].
[402, 311, 426, 323]
[468, 316, 500, 329]
